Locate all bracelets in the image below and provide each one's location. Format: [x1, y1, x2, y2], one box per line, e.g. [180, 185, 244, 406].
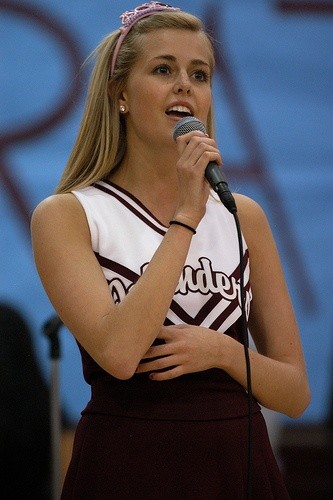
[169, 220, 197, 236]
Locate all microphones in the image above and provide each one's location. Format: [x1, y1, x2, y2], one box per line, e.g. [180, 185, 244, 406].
[171, 116, 238, 215]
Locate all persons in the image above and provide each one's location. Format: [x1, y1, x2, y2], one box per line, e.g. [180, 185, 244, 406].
[31, 2, 312, 500]
